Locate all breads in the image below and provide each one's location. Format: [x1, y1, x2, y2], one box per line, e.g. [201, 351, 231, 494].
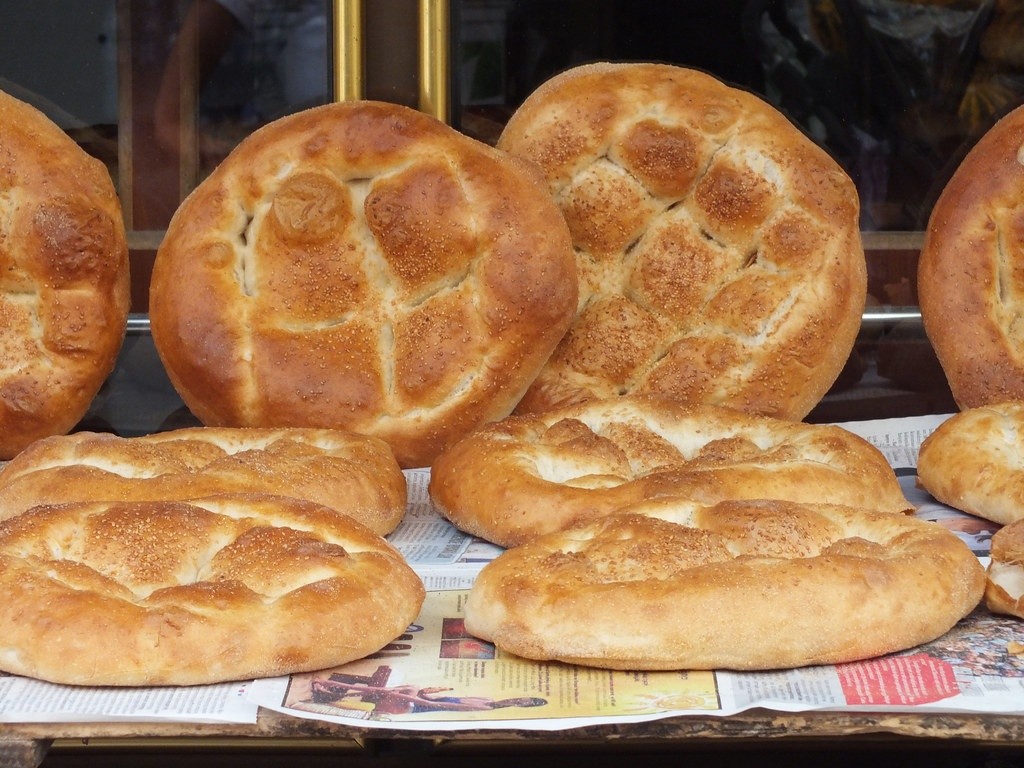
[427, 401, 1024, 673]
[495, 59, 867, 424]
[0, 93, 129, 464]
[150, 99, 578, 471]
[0, 425, 427, 684]
[917, 102, 1024, 412]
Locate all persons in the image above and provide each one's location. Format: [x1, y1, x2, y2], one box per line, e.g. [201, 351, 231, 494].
[155, 0, 328, 153]
